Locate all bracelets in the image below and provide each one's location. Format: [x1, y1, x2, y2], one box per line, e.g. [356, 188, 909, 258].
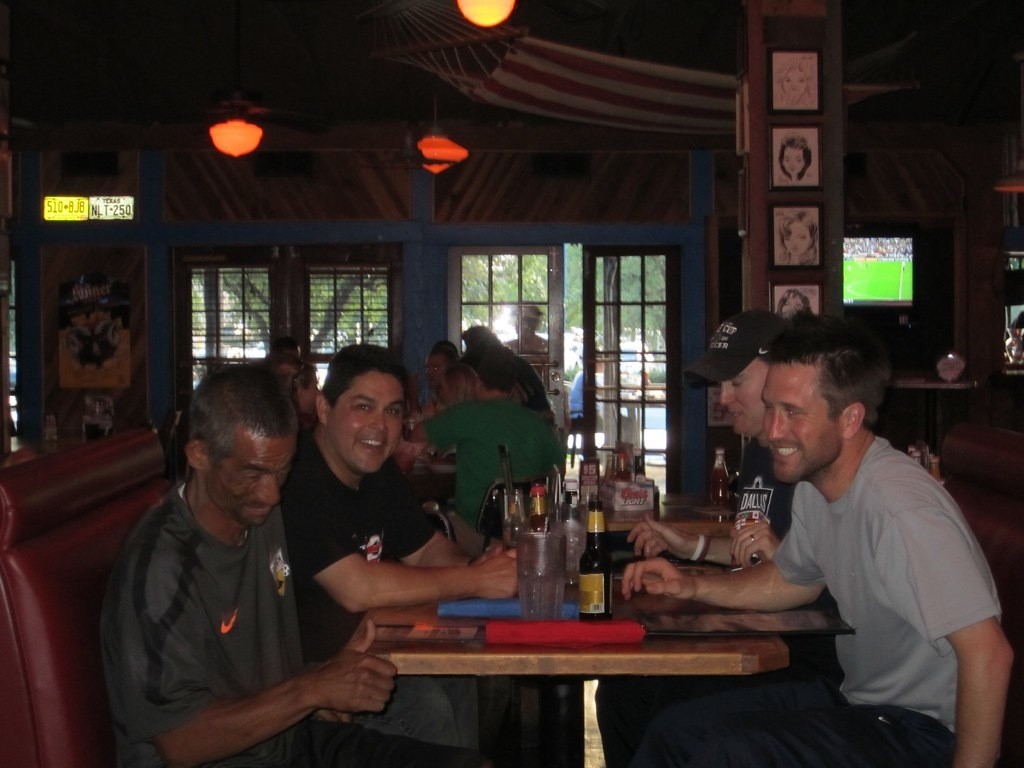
[691, 533, 705, 561]
[697, 535, 711, 561]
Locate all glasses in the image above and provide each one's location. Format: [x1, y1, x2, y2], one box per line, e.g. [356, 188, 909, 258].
[292, 360, 307, 393]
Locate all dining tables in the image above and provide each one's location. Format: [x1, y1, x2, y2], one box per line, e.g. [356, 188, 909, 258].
[889, 366, 978, 454]
[349, 578, 791, 768]
[604, 512, 739, 534]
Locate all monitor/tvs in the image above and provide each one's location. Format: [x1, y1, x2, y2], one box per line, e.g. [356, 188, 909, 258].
[842, 235, 914, 308]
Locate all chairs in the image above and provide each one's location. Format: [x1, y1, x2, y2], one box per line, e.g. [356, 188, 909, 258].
[940, 424, 1024, 768]
[0, 428, 172, 768]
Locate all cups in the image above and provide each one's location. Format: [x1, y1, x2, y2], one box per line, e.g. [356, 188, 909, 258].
[549, 503, 589, 570]
[513, 530, 567, 619]
[579, 501, 613, 622]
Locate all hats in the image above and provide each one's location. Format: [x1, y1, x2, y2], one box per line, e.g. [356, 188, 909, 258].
[683, 309, 786, 388]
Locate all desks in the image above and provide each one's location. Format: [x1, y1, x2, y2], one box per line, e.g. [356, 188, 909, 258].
[595, 388, 666, 449]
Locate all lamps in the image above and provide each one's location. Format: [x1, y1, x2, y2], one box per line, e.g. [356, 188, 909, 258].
[456, 0, 516, 28]
[206, 88, 264, 157]
[411, 122, 469, 175]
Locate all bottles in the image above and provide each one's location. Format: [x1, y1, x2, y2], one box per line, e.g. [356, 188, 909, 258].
[710, 448, 729, 508]
[905, 442, 939, 485]
[500, 451, 637, 546]
[407, 418, 416, 439]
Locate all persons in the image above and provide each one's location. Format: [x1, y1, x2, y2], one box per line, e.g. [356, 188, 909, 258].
[101, 366, 494, 768]
[568, 309, 1014, 768]
[265, 327, 565, 746]
[508, 305, 548, 384]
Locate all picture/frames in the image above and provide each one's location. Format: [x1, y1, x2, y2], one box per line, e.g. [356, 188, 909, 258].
[769, 281, 824, 316]
[768, 123, 824, 191]
[769, 48, 823, 114]
[768, 201, 824, 271]
